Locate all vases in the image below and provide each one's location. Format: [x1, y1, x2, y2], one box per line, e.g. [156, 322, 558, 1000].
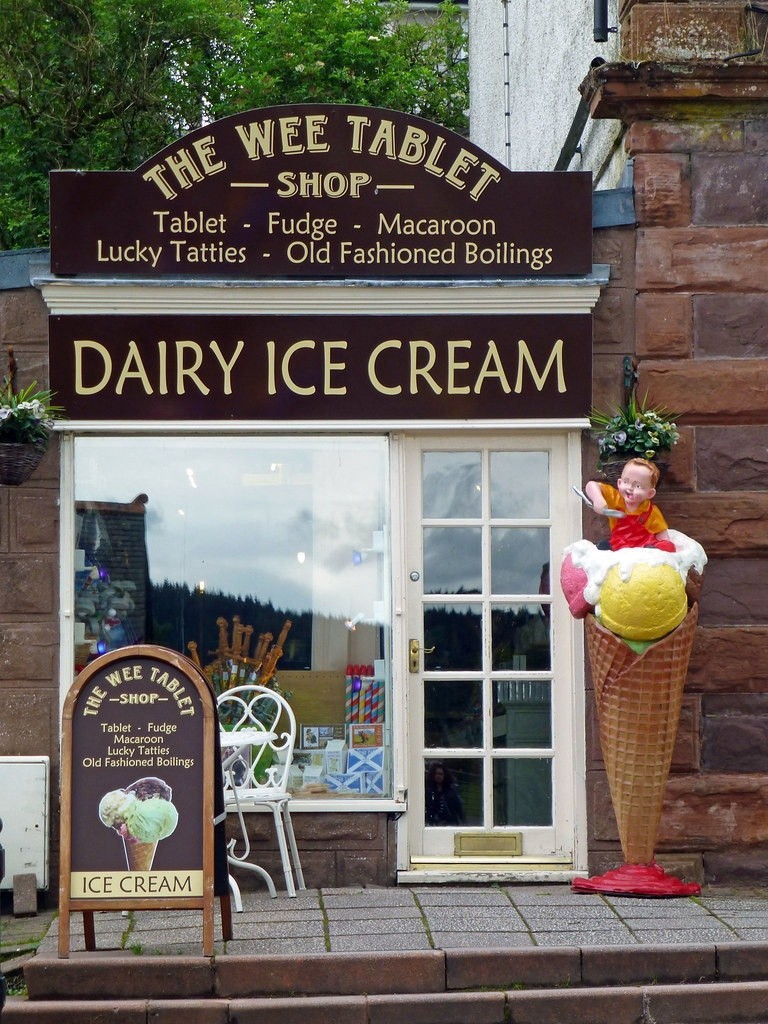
[604, 461, 669, 489]
[0, 443, 47, 486]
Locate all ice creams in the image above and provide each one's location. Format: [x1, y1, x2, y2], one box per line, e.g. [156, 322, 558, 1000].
[560, 527, 708, 863]
[99, 778, 178, 871]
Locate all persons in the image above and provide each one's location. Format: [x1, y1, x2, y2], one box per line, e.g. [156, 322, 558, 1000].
[425, 764, 465, 827]
[586, 458, 676, 552]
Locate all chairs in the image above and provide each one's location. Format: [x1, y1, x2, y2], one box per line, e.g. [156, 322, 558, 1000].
[216, 685, 306, 899]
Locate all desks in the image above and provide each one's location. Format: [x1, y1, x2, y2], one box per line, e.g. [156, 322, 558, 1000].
[218, 732, 277, 911]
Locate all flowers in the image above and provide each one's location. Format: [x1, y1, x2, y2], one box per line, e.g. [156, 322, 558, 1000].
[582, 386, 686, 471]
[0, 375, 71, 445]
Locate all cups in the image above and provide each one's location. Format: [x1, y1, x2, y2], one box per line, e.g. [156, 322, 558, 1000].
[74, 549, 85, 572]
[74, 623, 85, 645]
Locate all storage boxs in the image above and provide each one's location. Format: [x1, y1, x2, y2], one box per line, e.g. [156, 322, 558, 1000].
[268, 724, 384, 794]
[495, 655, 552, 701]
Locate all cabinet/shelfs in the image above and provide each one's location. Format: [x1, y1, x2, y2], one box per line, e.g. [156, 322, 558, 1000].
[493, 701, 551, 824]
